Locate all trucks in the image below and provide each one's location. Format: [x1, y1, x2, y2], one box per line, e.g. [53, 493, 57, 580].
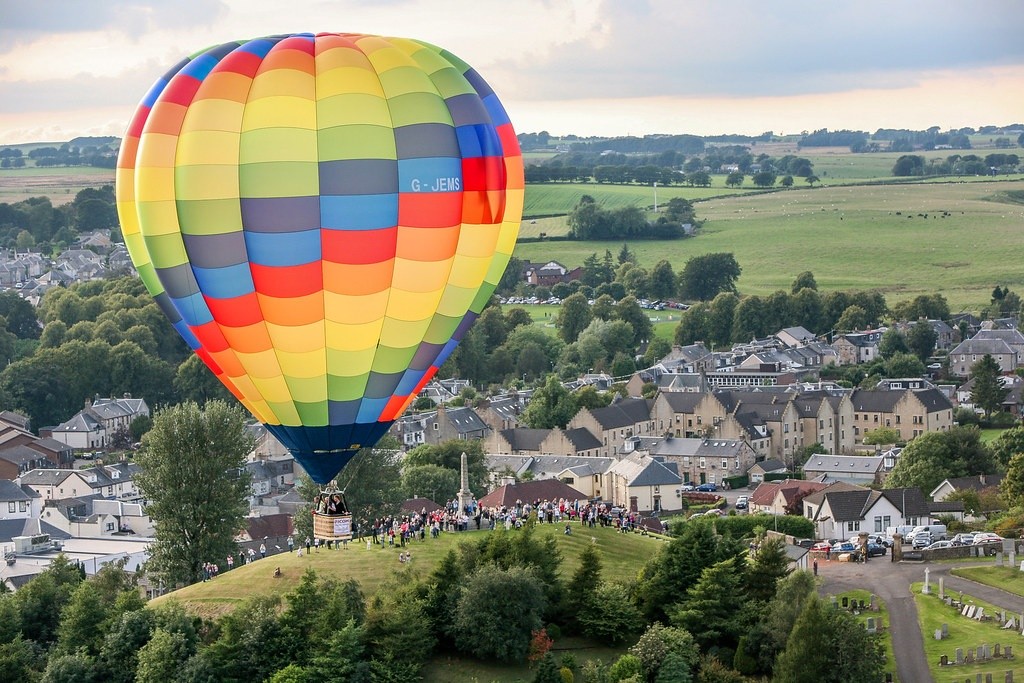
[906, 524, 948, 544]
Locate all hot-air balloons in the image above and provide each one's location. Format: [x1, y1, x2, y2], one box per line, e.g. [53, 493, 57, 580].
[116, 31, 524, 541]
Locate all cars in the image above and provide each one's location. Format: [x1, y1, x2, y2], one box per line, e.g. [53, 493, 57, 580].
[694, 483, 717, 492]
[682, 481, 694, 491]
[806, 531, 1004, 558]
[735, 496, 748, 509]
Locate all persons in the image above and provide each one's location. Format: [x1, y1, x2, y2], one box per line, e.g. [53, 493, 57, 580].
[200, 492, 648, 583]
[813, 558, 819, 577]
[826, 545, 831, 560]
[876, 536, 882, 544]
